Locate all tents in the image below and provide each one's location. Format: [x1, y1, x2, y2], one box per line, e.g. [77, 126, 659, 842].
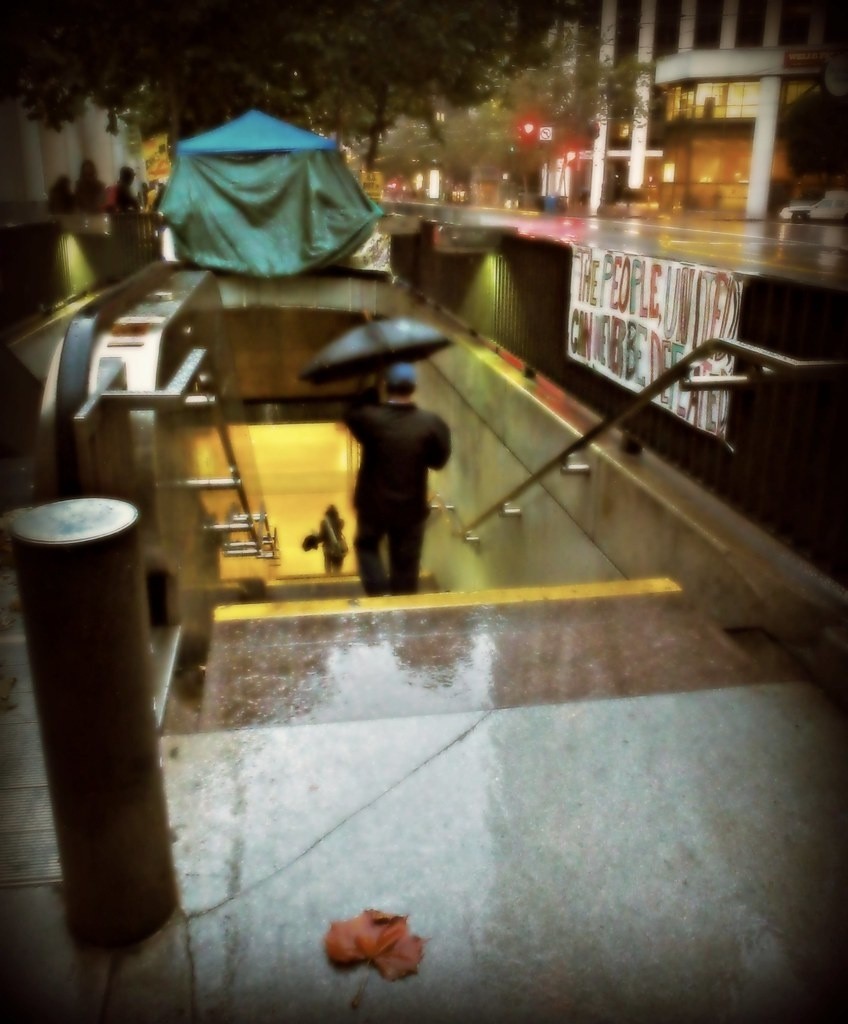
[158, 110, 383, 281]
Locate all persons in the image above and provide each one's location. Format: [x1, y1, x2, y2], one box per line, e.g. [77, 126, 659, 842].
[316, 504, 347, 573]
[138, 179, 165, 210]
[77, 161, 103, 211]
[342, 362, 451, 595]
[115, 167, 140, 210]
[47, 179, 77, 215]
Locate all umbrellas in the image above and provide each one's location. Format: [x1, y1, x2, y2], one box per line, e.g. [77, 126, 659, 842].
[303, 535, 318, 551]
[300, 310, 452, 398]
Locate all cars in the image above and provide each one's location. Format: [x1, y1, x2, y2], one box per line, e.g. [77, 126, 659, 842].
[779, 197, 848, 225]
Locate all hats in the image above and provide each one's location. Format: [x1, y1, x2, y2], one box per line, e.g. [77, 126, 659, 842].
[385, 362, 416, 386]
